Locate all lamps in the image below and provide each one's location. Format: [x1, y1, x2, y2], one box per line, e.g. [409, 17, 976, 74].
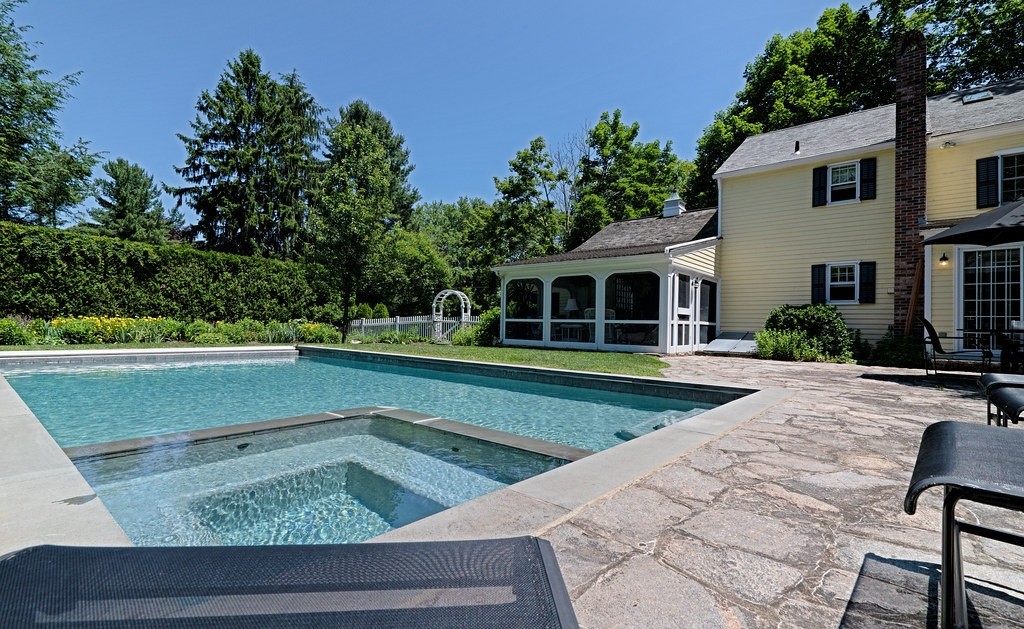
[940, 253, 948, 265]
[564, 299, 579, 324]
[692, 280, 699, 289]
[940, 141, 956, 150]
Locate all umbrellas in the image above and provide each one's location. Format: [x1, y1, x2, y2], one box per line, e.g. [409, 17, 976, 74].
[918, 196, 1024, 246]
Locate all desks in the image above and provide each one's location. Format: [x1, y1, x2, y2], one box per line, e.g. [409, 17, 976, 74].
[560, 324, 583, 342]
[956, 328, 1024, 373]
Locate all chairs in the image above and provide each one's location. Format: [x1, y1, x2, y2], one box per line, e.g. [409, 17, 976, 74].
[535, 316, 561, 341]
[0, 535, 582, 629]
[904, 373, 1024, 629]
[584, 308, 622, 344]
[915, 316, 993, 378]
